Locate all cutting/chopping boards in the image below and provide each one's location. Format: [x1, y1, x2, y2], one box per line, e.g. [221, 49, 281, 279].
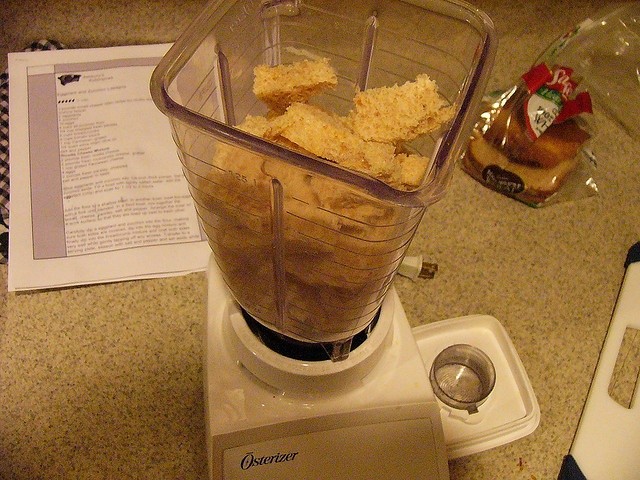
[556, 238, 640, 480]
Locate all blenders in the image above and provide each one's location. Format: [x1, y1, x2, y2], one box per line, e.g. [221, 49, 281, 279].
[148, 0, 540, 480]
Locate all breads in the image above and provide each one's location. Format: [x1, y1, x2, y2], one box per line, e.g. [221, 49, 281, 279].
[461, 80, 590, 210]
